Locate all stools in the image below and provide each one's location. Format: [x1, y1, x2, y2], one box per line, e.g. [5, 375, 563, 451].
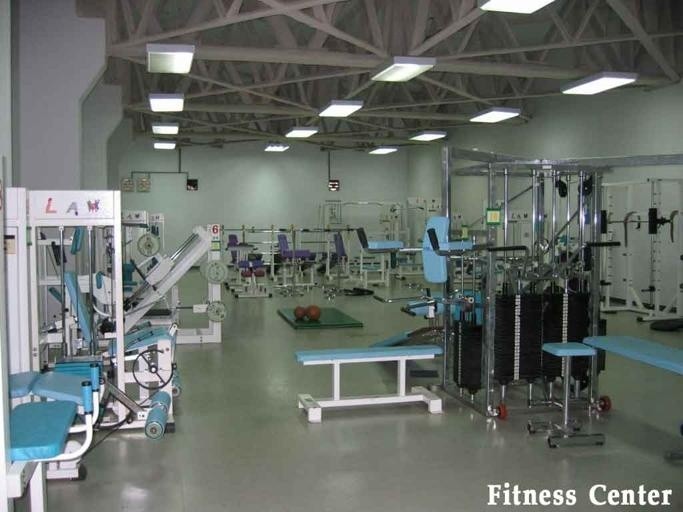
[527, 342, 605, 447]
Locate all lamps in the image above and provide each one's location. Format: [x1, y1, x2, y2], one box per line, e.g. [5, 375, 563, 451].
[146, 45, 193, 151]
